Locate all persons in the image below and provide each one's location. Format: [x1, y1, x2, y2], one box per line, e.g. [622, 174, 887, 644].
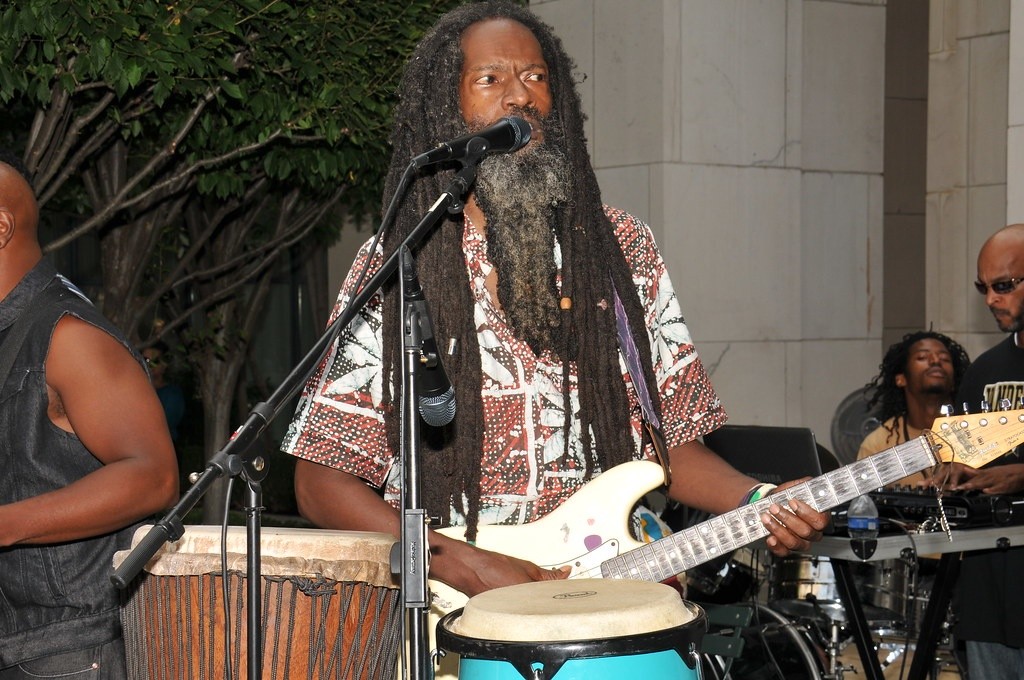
[0, 163, 183, 680]
[281, 2, 831, 600]
[917, 224, 1024, 679]
[858, 328, 971, 489]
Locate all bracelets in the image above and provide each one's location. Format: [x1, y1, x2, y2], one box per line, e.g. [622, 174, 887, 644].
[738, 483, 778, 510]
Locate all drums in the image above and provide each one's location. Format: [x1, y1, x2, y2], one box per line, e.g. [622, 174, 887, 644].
[435, 576, 710, 680]
[110, 521, 401, 680]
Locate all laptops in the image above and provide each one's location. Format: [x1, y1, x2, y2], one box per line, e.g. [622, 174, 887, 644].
[703, 425, 918, 537]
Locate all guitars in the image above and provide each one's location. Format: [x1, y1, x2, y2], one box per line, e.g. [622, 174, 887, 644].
[396, 394, 1024, 680]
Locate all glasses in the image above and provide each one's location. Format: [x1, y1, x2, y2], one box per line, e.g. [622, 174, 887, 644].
[974, 276, 1024, 296]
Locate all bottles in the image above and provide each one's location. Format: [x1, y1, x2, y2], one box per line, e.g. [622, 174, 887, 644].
[847, 493, 879, 538]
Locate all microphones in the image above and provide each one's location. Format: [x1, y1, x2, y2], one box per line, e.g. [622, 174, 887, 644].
[403, 244, 456, 426]
[412, 116, 532, 169]
